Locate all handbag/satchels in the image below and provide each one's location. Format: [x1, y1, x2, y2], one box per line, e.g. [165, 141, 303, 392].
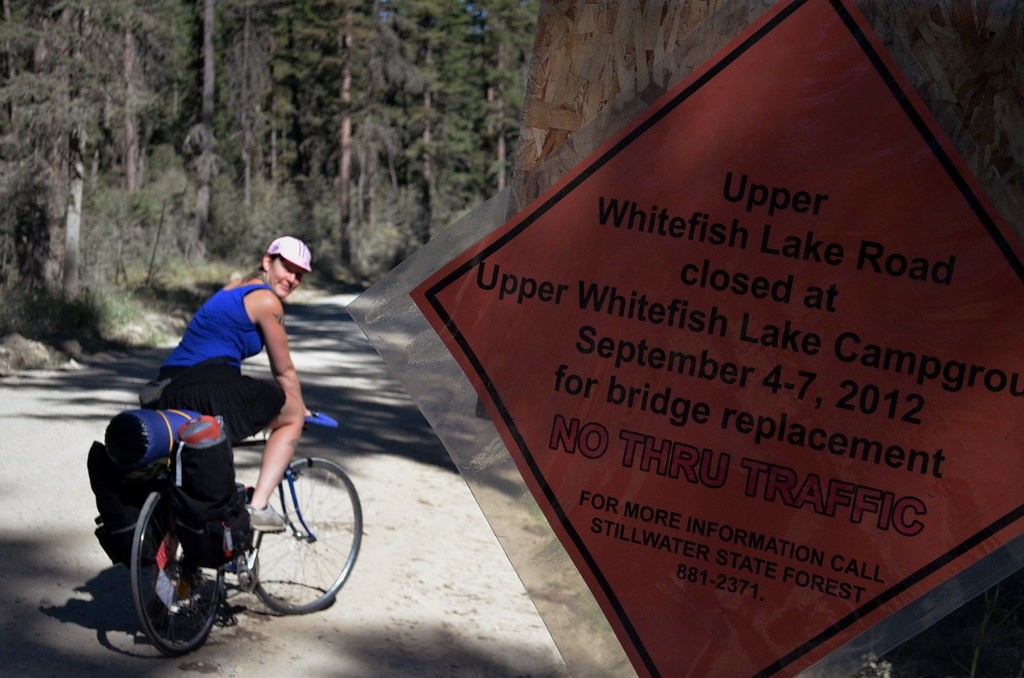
[140, 356, 240, 406]
[88, 409, 239, 574]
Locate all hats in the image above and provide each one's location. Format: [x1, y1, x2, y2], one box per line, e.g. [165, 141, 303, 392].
[267, 236, 312, 272]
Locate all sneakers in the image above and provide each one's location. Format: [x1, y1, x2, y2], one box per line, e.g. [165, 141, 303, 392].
[242, 503, 286, 532]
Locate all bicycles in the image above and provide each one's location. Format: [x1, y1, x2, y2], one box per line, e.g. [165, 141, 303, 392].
[130, 410, 363, 655]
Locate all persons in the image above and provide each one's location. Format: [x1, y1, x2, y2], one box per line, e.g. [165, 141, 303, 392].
[140, 236, 314, 629]
